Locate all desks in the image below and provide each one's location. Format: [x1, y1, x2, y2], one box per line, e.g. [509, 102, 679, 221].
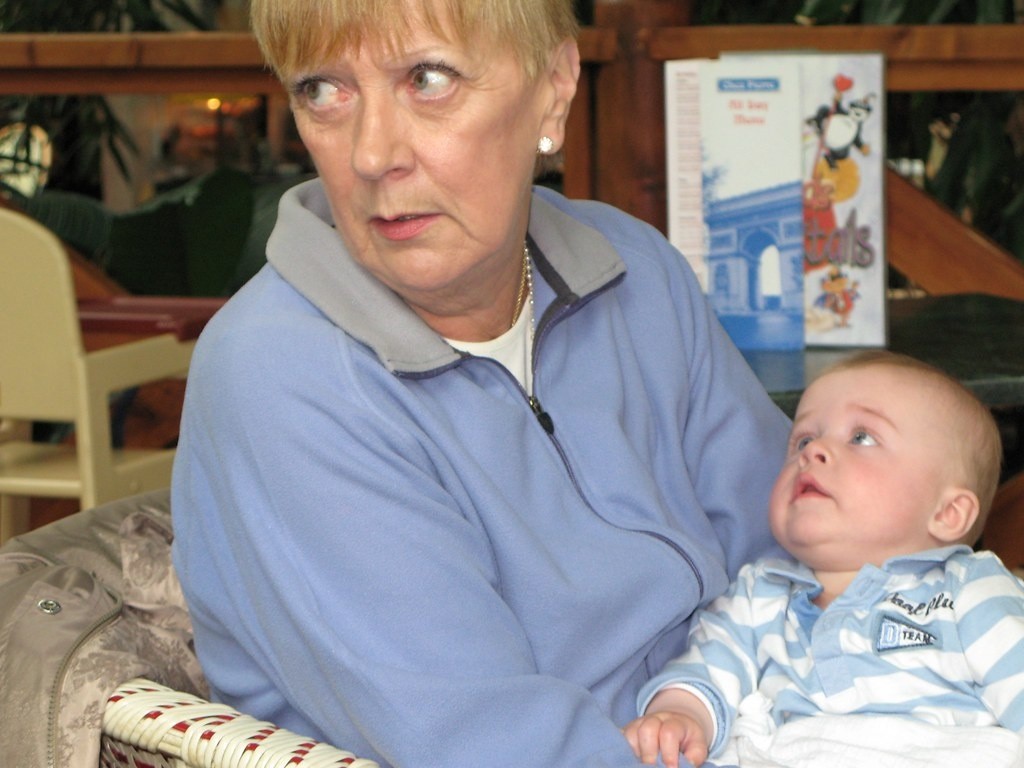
[746, 292, 1024, 411]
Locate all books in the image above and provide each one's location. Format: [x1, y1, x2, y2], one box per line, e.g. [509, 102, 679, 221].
[665, 51, 892, 344]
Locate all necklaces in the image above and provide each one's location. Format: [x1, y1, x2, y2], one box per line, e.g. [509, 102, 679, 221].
[512, 239, 536, 345]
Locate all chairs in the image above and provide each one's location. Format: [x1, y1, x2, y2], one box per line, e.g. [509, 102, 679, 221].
[1, 207, 195, 541]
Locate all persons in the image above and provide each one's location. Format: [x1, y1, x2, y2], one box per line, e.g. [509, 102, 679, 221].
[621, 350, 1024, 768]
[181, 2, 804, 767]
